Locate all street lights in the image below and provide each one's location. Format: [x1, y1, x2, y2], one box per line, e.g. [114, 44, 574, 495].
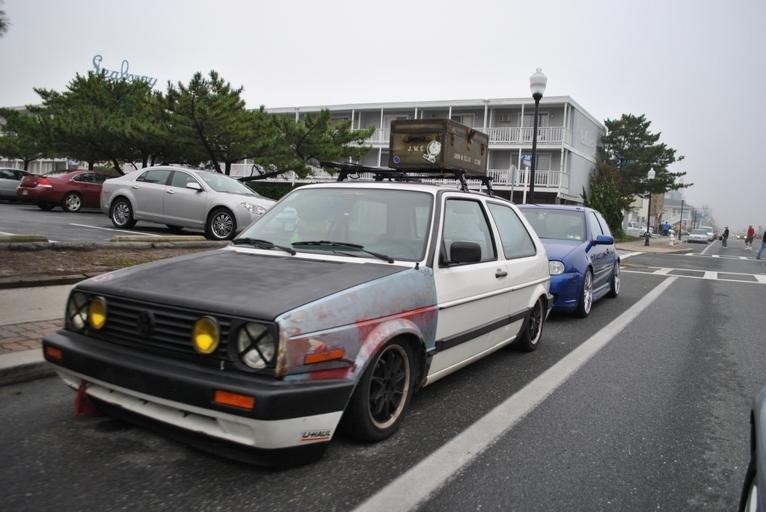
[529, 67, 547, 203]
[644, 167, 656, 246]
[678, 195, 685, 240]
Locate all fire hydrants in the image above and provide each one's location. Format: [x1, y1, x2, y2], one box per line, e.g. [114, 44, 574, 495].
[668, 229, 676, 247]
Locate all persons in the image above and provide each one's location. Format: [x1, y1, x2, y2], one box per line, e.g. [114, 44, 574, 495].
[743, 223, 755, 251]
[661, 220, 670, 235]
[673, 220, 680, 239]
[721, 226, 729, 249]
[755, 229, 766, 259]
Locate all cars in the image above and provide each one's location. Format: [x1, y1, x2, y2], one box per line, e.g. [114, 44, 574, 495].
[688, 226, 717, 244]
[736, 232, 747, 240]
[622, 221, 653, 237]
[739, 384, 766, 512]
